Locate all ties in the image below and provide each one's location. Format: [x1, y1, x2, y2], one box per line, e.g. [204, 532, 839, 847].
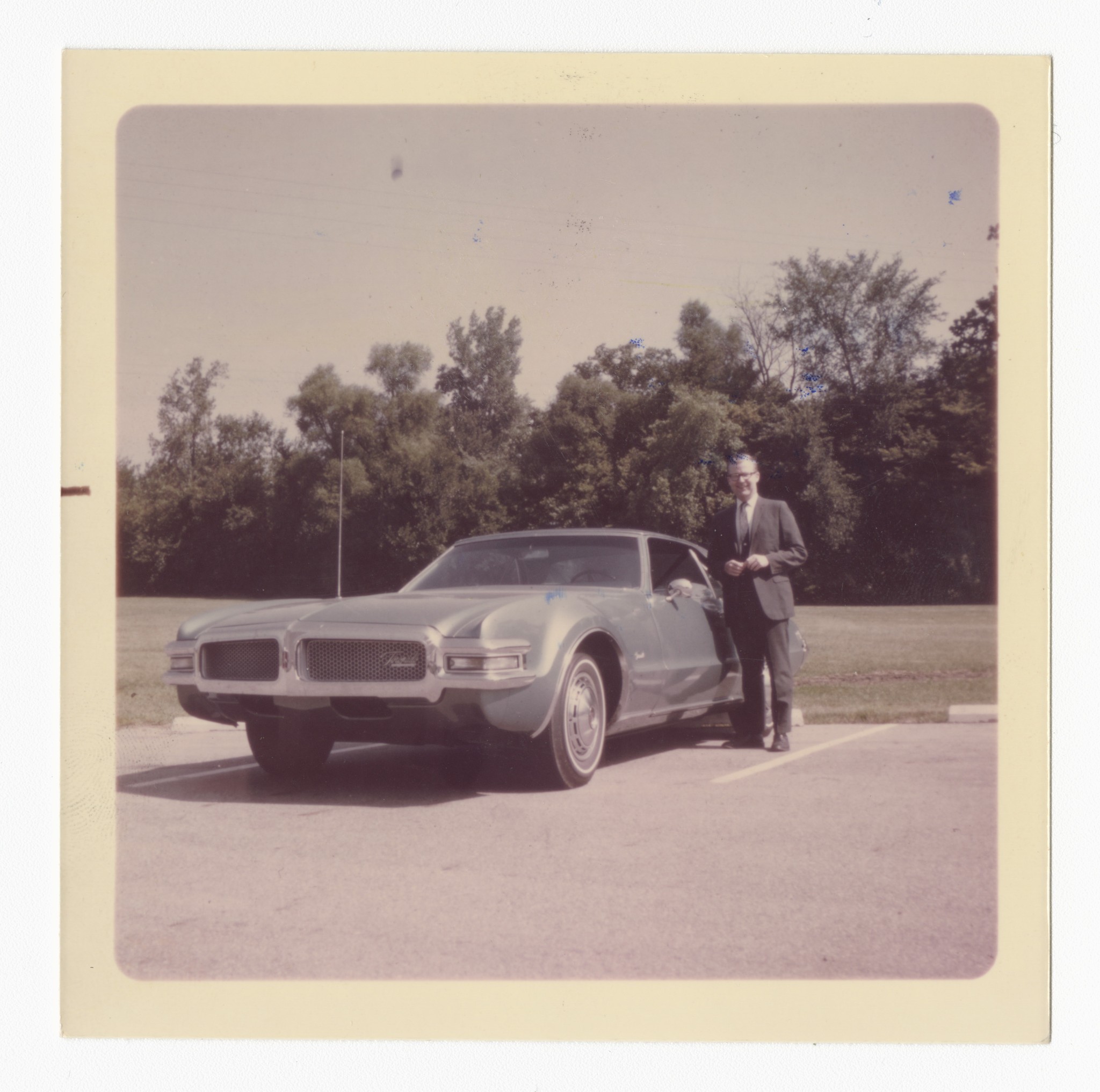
[739, 502, 751, 562]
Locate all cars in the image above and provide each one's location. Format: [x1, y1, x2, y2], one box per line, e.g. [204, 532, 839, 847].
[164, 527, 812, 791]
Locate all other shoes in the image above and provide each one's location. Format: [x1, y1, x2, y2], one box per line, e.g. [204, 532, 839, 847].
[722, 731, 764, 750]
[770, 734, 790, 752]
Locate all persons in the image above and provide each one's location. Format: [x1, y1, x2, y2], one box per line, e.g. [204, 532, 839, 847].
[707, 454, 808, 752]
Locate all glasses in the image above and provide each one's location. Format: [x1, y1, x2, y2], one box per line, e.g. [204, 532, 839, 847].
[726, 470, 758, 481]
